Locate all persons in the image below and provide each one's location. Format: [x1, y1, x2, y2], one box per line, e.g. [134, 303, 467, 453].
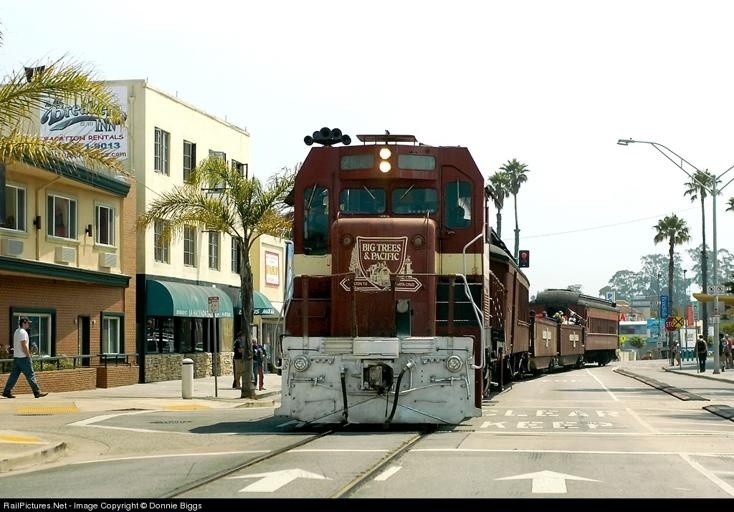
[1, 316, 49, 399]
[0, 215, 16, 229]
[251, 337, 267, 391]
[694, 334, 708, 373]
[718, 333, 729, 372]
[731, 335, 734, 369]
[675, 340, 682, 366]
[725, 333, 732, 369]
[669, 341, 677, 366]
[528, 309, 583, 327]
[232, 330, 242, 390]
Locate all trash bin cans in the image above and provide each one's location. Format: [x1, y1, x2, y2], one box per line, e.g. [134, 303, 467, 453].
[661, 350, 671, 359]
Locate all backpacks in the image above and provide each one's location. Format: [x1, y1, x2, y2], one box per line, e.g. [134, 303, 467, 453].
[697, 339, 706, 352]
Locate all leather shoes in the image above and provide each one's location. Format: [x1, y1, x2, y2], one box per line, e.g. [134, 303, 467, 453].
[2, 392, 16, 398]
[34, 392, 48, 398]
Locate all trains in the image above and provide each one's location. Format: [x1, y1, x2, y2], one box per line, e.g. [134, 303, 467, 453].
[274, 127, 619, 424]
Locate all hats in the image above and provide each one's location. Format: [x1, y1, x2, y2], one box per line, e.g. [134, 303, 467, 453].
[20, 316, 33, 324]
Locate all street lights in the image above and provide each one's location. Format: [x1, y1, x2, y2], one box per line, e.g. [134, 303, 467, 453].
[617, 135, 734, 374]
